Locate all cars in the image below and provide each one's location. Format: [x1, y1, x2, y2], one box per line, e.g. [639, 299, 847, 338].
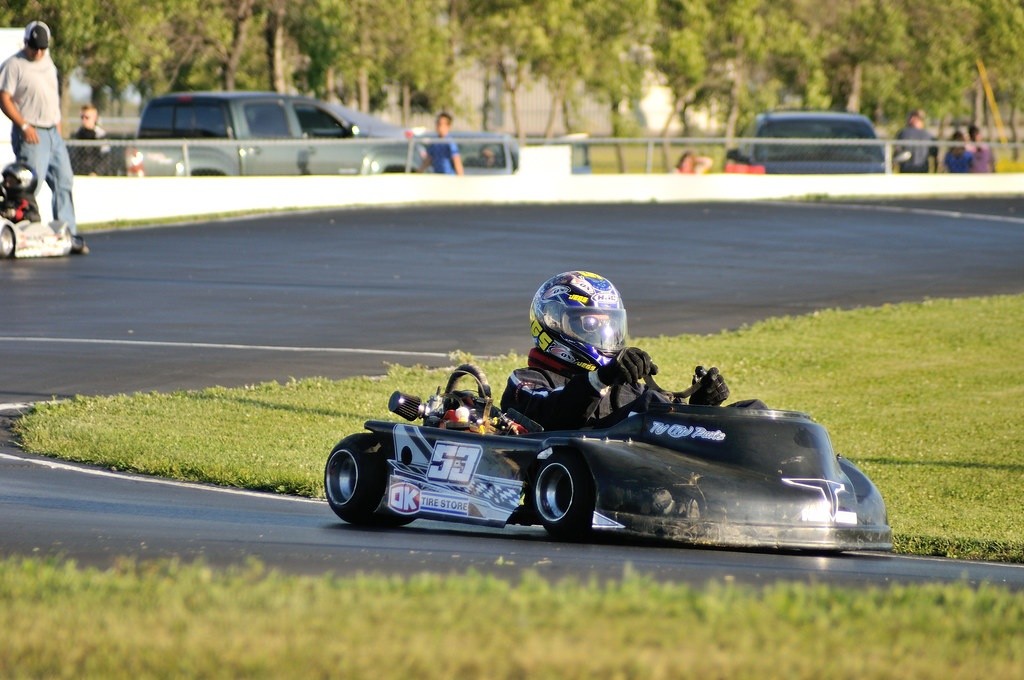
[726, 111, 888, 175]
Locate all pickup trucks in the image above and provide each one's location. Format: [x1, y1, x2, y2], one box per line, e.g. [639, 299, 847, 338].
[126, 84, 422, 178]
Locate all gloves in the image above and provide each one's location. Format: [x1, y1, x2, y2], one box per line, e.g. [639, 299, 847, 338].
[598, 347, 658, 387]
[689, 366, 730, 406]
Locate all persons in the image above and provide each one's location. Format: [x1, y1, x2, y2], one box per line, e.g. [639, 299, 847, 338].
[943, 122, 996, 173]
[66, 102, 110, 177]
[725, 149, 766, 174]
[894, 108, 939, 173]
[0, 162, 42, 223]
[670, 151, 713, 175]
[500, 271, 729, 432]
[416, 113, 463, 175]
[0, 22, 89, 255]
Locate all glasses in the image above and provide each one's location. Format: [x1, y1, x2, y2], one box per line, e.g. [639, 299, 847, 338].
[570, 315, 618, 333]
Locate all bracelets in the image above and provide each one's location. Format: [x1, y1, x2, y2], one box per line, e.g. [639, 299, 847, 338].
[21, 122, 30, 130]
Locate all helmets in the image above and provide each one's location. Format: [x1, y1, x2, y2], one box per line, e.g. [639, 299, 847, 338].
[530, 271, 628, 375]
[2, 164, 37, 193]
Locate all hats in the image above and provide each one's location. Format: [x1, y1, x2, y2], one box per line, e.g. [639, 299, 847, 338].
[26, 21, 51, 48]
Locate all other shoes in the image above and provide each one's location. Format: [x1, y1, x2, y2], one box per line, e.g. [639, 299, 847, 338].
[72, 236, 89, 255]
